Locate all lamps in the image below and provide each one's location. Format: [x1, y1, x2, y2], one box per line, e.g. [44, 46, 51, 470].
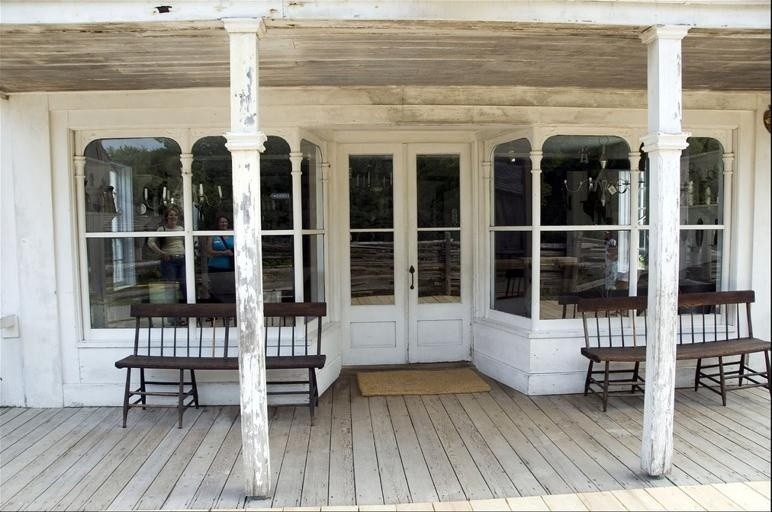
[140, 180, 225, 222]
[560, 136, 646, 208]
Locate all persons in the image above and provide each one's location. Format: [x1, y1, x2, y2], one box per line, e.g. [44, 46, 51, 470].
[146, 206, 186, 304]
[206, 214, 235, 273]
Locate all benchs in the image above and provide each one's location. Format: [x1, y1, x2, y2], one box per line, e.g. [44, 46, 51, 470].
[556, 281, 718, 320]
[572, 287, 772, 410]
[111, 300, 327, 429]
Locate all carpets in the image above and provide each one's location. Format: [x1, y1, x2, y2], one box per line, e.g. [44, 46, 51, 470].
[354, 364, 493, 399]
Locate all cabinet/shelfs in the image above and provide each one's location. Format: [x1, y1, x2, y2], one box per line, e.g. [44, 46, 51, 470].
[675, 147, 724, 289]
